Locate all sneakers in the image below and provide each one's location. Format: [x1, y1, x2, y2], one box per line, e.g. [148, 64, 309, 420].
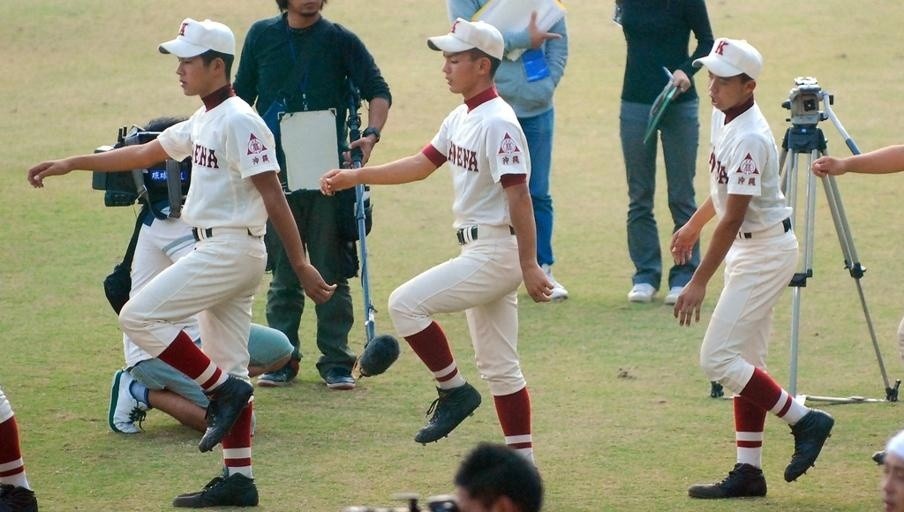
[258, 356, 299, 386]
[664, 286, 683, 305]
[321, 375, 355, 389]
[199, 374, 254, 453]
[414, 380, 481, 446]
[784, 408, 834, 482]
[688, 463, 767, 498]
[541, 263, 568, 299]
[173, 472, 258, 507]
[109, 369, 148, 434]
[628, 282, 656, 303]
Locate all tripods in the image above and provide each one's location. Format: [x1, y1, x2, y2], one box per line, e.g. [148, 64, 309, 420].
[710, 76, 902, 404]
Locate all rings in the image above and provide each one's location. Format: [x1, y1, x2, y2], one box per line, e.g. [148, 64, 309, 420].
[679, 86, 686, 93]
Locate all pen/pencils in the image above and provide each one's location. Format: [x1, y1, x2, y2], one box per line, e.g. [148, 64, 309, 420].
[662, 65, 675, 81]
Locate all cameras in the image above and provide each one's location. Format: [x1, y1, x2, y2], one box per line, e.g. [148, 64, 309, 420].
[348, 491, 462, 512]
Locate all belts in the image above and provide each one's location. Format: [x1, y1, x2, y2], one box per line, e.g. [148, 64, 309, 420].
[191, 227, 212, 241]
[456, 225, 515, 242]
[744, 217, 791, 238]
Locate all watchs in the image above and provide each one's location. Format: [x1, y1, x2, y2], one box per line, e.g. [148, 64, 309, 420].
[361, 127, 379, 143]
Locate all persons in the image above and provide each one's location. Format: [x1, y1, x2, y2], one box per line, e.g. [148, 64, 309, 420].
[27, 18, 339, 507]
[1, 390, 34, 510]
[454, 440, 543, 511]
[872, 428, 903, 511]
[809, 145, 903, 359]
[670, 38, 836, 498]
[232, 1, 392, 389]
[444, 1, 569, 302]
[613, 1, 715, 306]
[321, 16, 553, 469]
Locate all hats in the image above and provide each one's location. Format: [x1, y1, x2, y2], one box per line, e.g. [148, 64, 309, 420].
[427, 17, 504, 61]
[158, 16, 235, 58]
[692, 37, 762, 80]
